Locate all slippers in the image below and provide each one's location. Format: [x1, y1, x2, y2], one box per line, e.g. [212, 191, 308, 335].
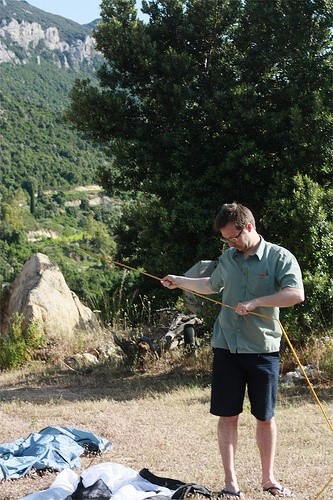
[263, 485, 285, 498]
[221, 489, 241, 500]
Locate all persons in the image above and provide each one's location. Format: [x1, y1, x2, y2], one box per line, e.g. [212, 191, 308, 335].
[161, 203, 306, 500]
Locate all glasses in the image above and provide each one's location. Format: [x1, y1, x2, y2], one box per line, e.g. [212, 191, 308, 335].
[220, 229, 242, 244]
